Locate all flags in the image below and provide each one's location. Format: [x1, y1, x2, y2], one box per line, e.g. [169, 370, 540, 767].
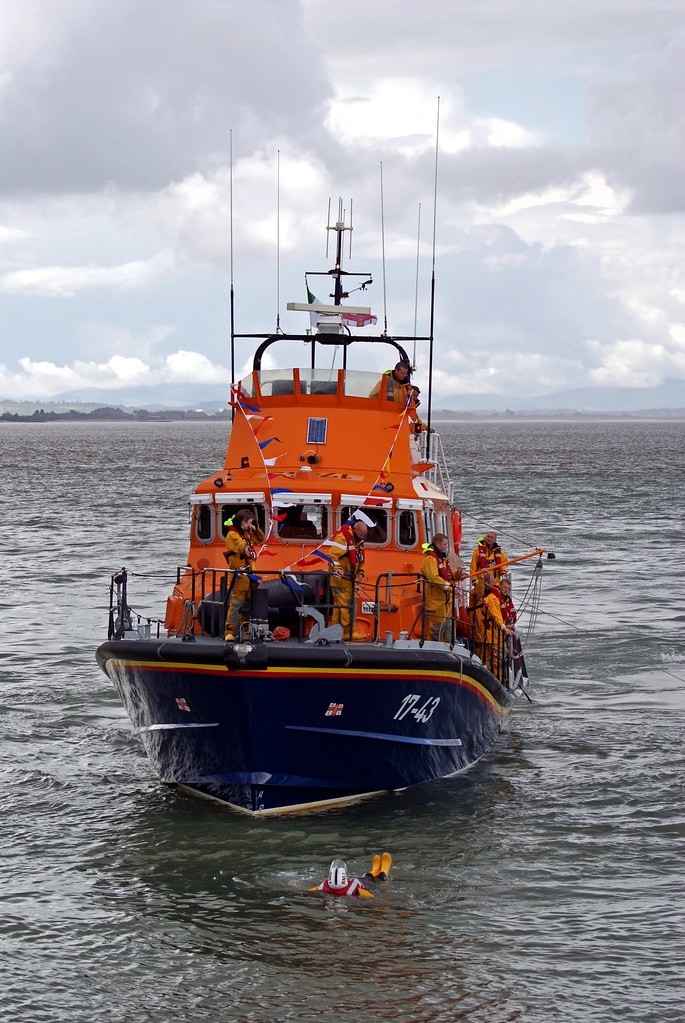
[308, 289, 377, 327]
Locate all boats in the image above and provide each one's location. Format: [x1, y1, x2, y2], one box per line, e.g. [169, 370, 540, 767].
[91, 93, 557, 819]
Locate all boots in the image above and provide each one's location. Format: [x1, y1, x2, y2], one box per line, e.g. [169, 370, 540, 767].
[369, 854, 381, 877]
[379, 852, 392, 877]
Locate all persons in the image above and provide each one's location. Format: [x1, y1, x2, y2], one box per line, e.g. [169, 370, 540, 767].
[305, 851, 391, 898]
[369, 361, 410, 403]
[223, 509, 264, 642]
[409, 385, 435, 433]
[327, 519, 368, 640]
[421, 533, 457, 642]
[475, 579, 517, 681]
[470, 530, 508, 605]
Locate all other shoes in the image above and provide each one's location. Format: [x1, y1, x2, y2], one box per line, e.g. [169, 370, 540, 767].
[342, 632, 367, 640]
[225, 634, 235, 641]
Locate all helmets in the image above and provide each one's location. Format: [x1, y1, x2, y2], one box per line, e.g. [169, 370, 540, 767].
[328, 867, 348, 889]
